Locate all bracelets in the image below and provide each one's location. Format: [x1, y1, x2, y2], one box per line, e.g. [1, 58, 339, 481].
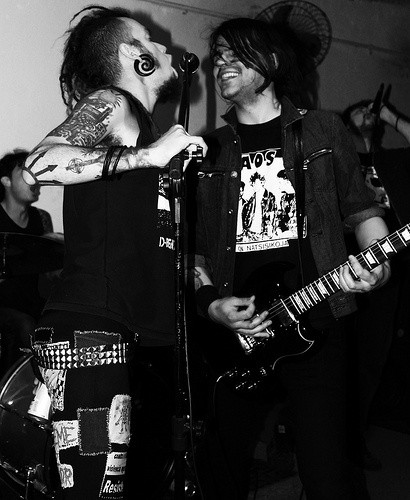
[395, 117, 400, 131]
[101, 145, 127, 179]
[194, 285, 222, 319]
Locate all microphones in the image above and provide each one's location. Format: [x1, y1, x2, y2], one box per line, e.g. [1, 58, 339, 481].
[178, 51, 200, 74]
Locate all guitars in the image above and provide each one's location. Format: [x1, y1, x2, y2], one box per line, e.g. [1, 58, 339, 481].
[188, 221, 410, 415]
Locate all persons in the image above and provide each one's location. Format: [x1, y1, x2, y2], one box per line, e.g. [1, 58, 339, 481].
[338, 99, 410, 469]
[0, 148, 64, 355]
[182, 16, 391, 500]
[22, 6, 210, 500]
